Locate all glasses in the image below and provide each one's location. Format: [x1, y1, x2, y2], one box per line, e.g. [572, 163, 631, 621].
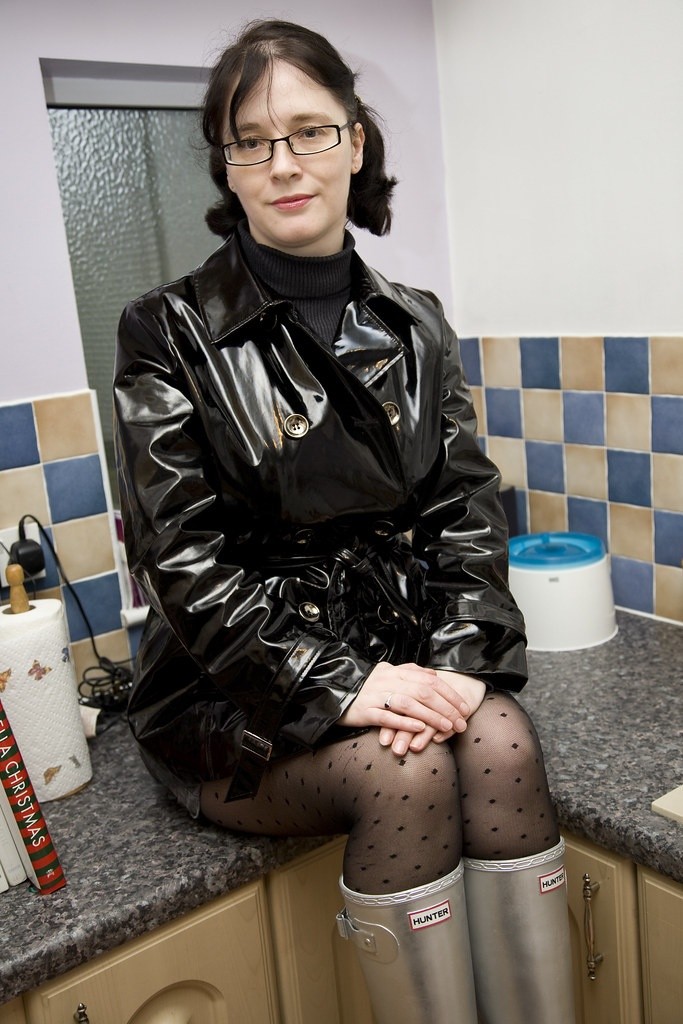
[220, 120, 350, 166]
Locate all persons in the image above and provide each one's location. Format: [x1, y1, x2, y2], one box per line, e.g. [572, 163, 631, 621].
[115, 15, 580, 1024]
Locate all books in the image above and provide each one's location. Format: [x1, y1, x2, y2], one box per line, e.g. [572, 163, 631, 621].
[1, 702, 68, 902]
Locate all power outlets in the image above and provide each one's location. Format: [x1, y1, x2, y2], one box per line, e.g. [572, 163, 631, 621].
[0, 521, 46, 588]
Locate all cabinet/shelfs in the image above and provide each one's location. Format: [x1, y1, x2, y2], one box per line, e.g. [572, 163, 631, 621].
[637, 860, 683, 1024]
[24, 879, 282, 1024]
[560, 836, 642, 1024]
[266, 833, 375, 1024]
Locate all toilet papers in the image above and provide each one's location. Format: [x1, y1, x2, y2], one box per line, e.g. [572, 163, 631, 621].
[2, 596, 94, 805]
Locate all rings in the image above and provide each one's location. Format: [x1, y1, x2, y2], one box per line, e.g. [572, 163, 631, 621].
[383, 691, 394, 710]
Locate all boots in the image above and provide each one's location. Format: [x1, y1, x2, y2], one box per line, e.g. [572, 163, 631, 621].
[463, 835, 576, 1024]
[336, 857, 478, 1024]
